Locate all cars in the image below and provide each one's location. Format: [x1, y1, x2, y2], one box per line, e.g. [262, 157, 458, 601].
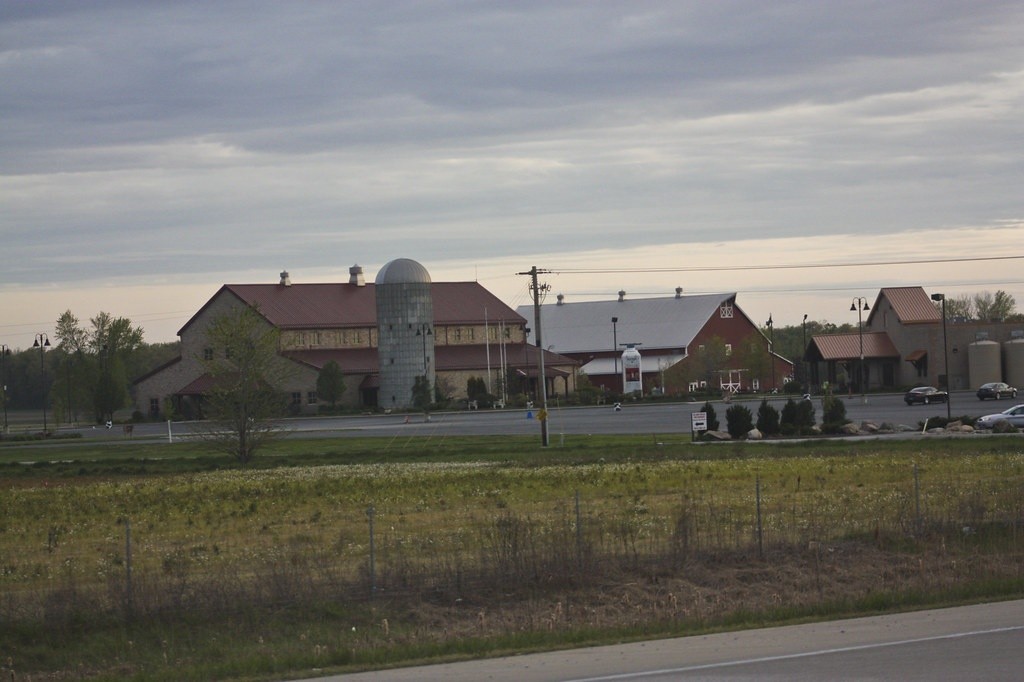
[976, 382, 1018, 401]
[976, 403, 1024, 431]
[904, 386, 949, 406]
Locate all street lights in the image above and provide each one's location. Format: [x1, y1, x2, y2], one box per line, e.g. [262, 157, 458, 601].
[33, 333, 51, 439]
[0, 344, 13, 433]
[611, 317, 619, 393]
[803, 314, 807, 393]
[416, 322, 433, 415]
[930, 293, 951, 420]
[766, 313, 775, 390]
[849, 297, 871, 396]
